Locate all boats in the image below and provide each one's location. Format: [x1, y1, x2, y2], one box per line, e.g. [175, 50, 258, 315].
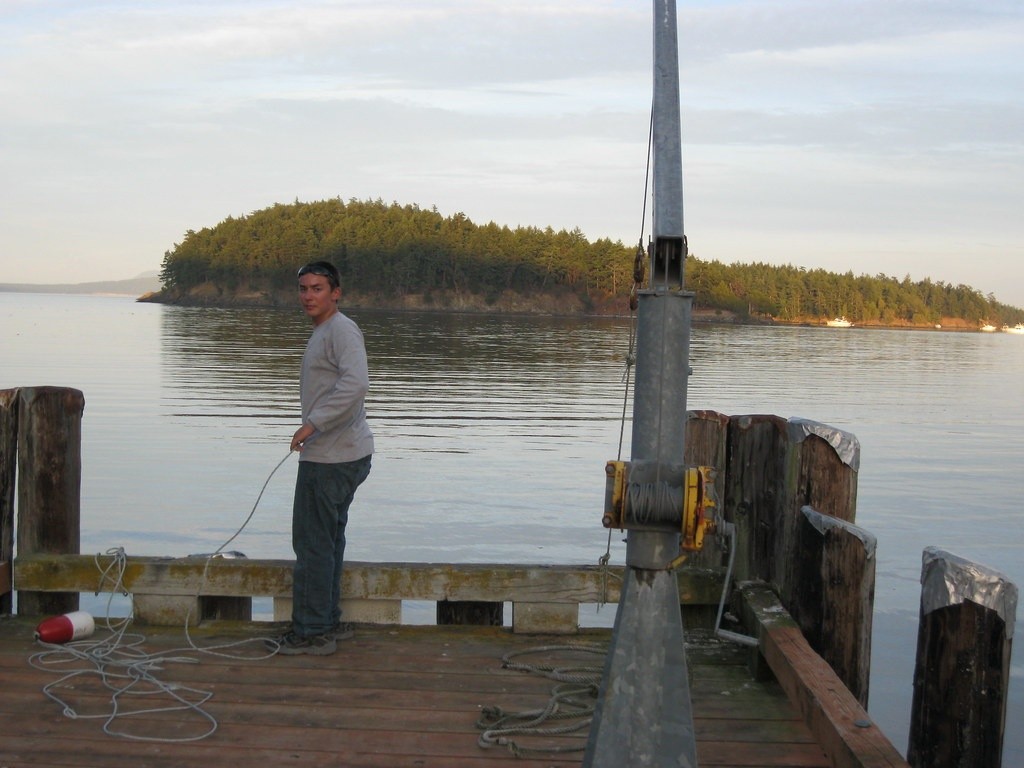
[979, 323, 997, 331]
[826, 316, 855, 327]
[1001, 321, 1024, 335]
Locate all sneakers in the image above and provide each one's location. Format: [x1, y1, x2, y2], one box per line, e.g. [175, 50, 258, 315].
[264, 627, 336, 654]
[334, 614, 355, 639]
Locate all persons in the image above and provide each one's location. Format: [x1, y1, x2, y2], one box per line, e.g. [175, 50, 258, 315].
[262, 261, 374, 655]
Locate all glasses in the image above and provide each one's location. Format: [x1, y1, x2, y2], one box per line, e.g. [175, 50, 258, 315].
[297, 265, 341, 287]
